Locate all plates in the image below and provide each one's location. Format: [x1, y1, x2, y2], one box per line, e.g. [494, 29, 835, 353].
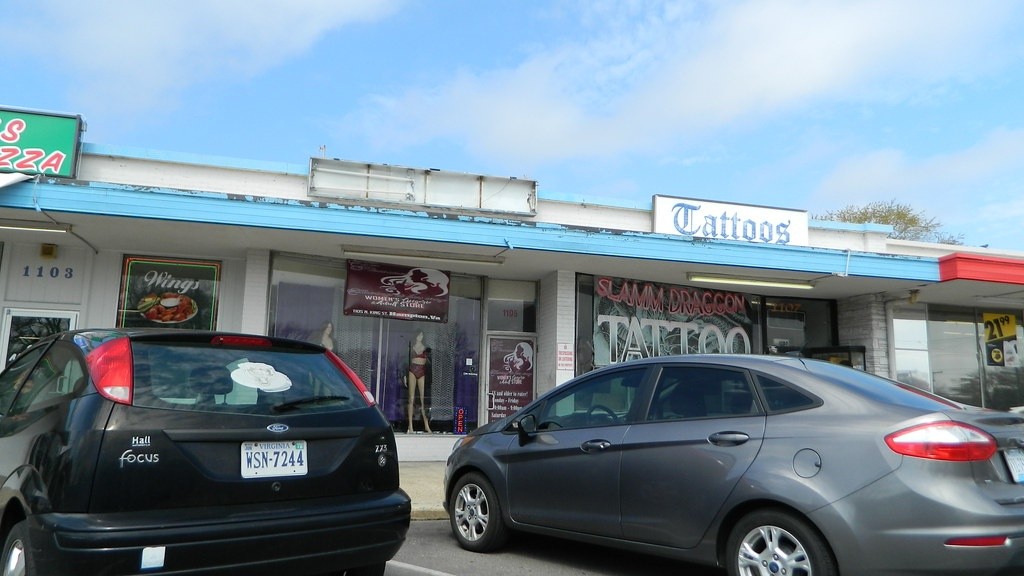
[140, 292, 198, 323]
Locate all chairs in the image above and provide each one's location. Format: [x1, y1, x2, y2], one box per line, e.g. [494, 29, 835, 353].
[189, 365, 233, 406]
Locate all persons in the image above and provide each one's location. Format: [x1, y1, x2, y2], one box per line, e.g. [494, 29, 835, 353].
[403, 329, 432, 433]
[308, 320, 338, 397]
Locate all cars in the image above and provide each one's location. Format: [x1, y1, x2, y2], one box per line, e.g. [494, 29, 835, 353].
[2, 325, 411, 575]
[443, 352, 1024, 575]
